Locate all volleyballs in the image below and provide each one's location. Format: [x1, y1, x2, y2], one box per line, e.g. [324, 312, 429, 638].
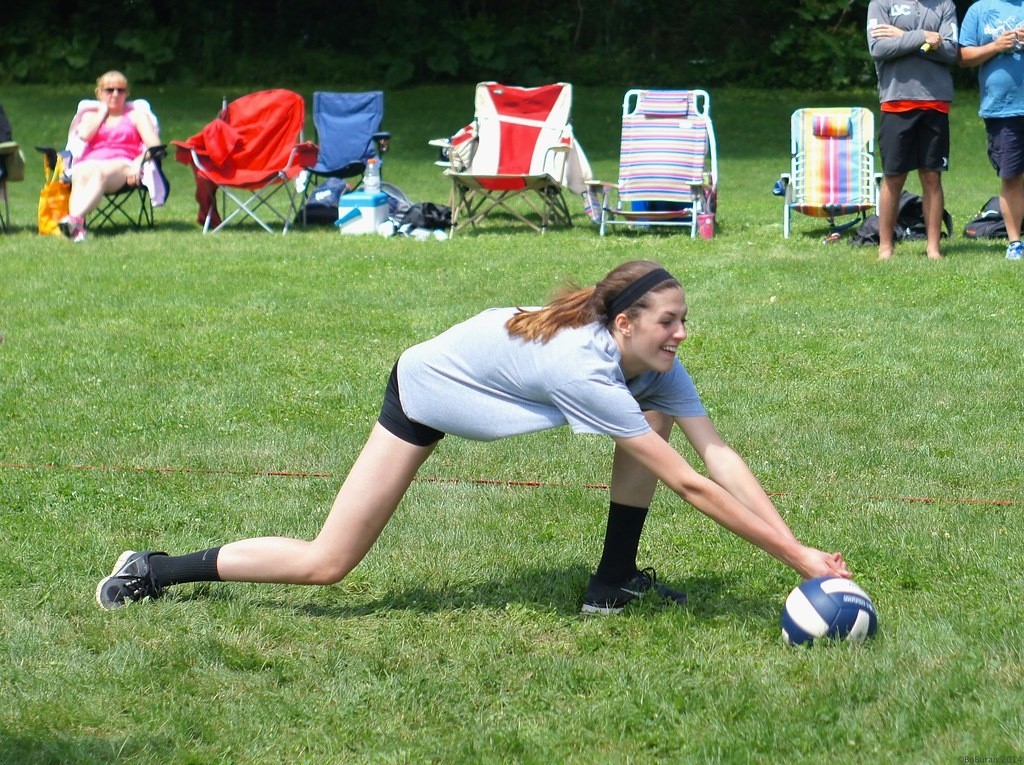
[780, 575, 880, 658]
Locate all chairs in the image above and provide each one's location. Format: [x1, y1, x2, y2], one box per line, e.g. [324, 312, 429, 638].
[584, 90, 716, 240]
[428, 81, 573, 235]
[171, 87, 317, 234]
[36, 99, 167, 230]
[298, 91, 389, 213]
[773, 106, 885, 239]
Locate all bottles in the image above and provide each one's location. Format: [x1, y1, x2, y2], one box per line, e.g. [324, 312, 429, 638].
[363, 158, 381, 195]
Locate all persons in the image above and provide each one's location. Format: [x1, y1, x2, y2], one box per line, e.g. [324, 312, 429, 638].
[57, 70, 161, 244]
[94, 259, 853, 615]
[958, 0, 1024, 262]
[867, 0, 958, 263]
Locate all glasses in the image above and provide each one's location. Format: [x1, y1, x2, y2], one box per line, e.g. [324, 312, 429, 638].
[104, 88, 124, 94]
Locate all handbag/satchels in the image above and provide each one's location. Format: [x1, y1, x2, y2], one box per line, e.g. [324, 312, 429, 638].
[853, 190, 954, 247]
[963, 195, 1024, 241]
[37, 156, 72, 238]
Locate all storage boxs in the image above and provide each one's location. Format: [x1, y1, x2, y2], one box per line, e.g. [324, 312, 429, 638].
[335, 192, 388, 234]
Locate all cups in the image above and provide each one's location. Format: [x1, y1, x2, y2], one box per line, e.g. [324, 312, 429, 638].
[698, 212, 714, 240]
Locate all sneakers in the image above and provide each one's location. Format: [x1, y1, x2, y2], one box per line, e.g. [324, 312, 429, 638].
[1005, 241, 1024, 261]
[581, 567, 693, 618]
[72, 228, 87, 243]
[96, 550, 169, 614]
[57, 215, 84, 237]
